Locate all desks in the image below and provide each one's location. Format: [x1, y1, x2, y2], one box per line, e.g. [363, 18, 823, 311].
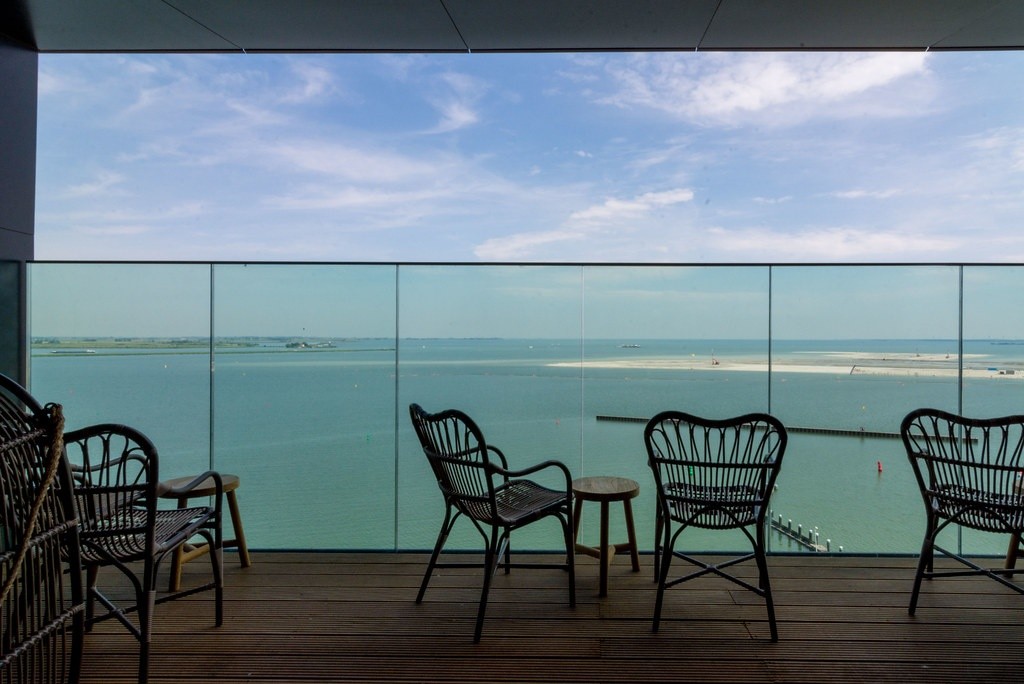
[158, 474, 251, 591]
[569, 475, 640, 600]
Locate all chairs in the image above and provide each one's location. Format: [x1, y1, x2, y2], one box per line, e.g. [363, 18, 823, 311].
[645, 412, 788, 642]
[900, 407, 1024, 621]
[408, 403, 576, 646]
[23, 422, 223, 684]
